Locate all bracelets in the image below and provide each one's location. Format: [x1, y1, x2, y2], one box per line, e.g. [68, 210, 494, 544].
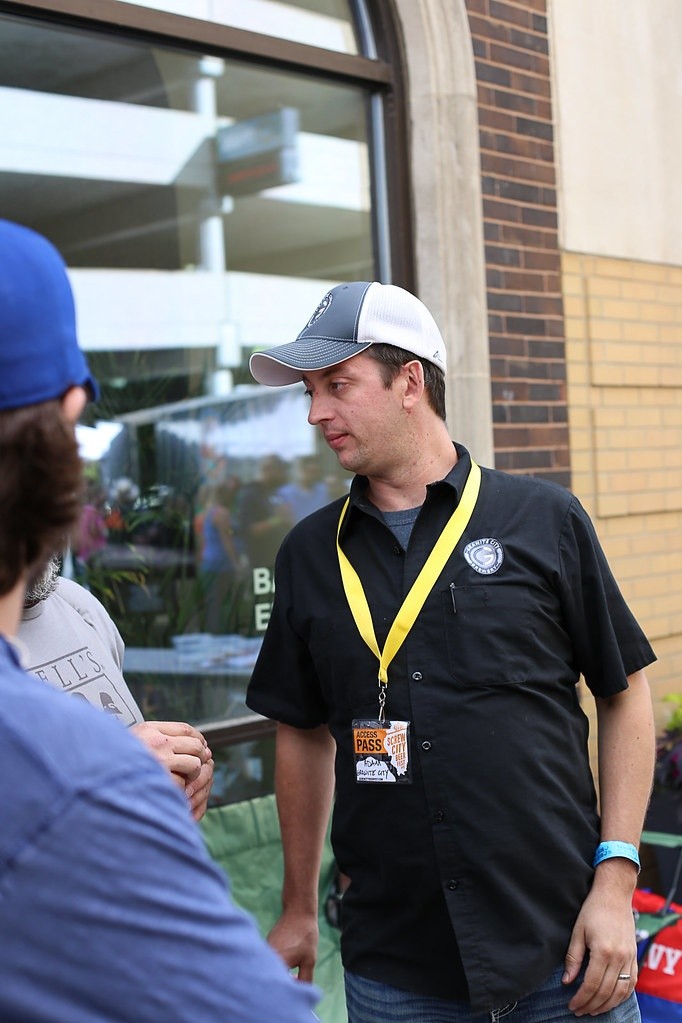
[592, 840, 642, 873]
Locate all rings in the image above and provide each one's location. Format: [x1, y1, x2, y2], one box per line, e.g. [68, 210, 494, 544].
[618, 975, 631, 979]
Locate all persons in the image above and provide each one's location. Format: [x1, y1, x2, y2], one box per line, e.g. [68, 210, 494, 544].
[12, 545, 213, 820]
[0, 218, 326, 1021]
[72, 449, 350, 636]
[250, 281, 660, 1022]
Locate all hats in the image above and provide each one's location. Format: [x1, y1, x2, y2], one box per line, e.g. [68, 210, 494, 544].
[0, 213, 98, 411]
[249, 282, 448, 386]
